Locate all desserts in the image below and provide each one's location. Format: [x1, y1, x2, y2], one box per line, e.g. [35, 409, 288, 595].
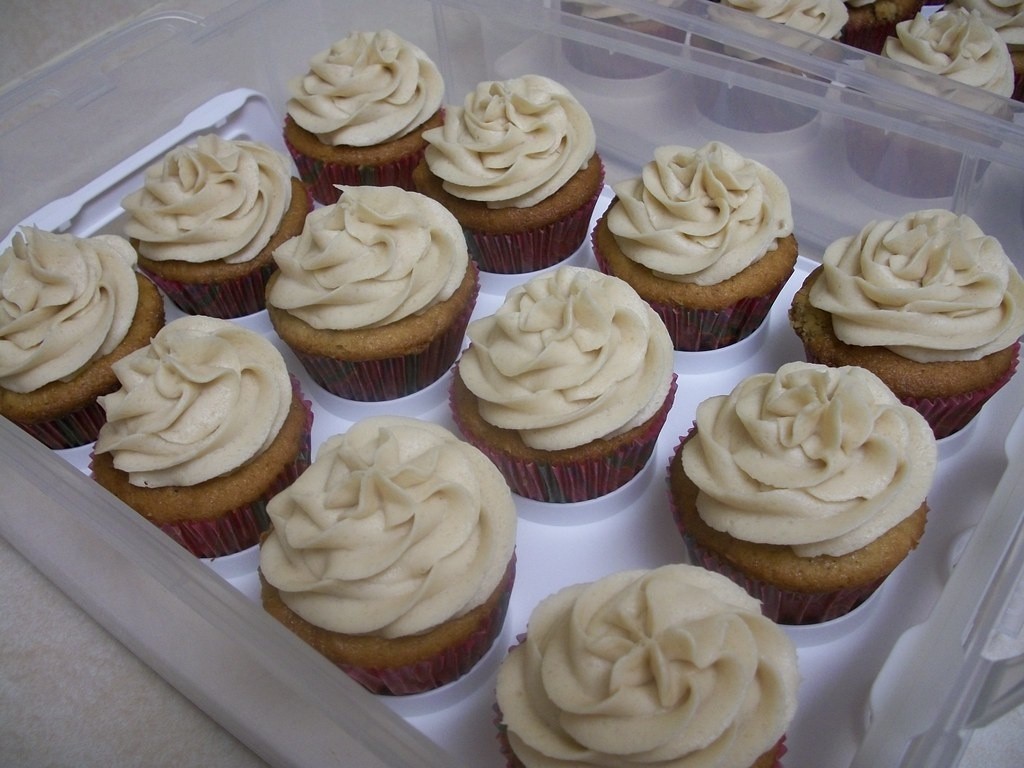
[0, 27, 1024, 768]
[548, 0, 1024, 198]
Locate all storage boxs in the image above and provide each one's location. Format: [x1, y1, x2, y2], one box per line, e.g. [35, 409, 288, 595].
[0, 0, 1022, 767]
